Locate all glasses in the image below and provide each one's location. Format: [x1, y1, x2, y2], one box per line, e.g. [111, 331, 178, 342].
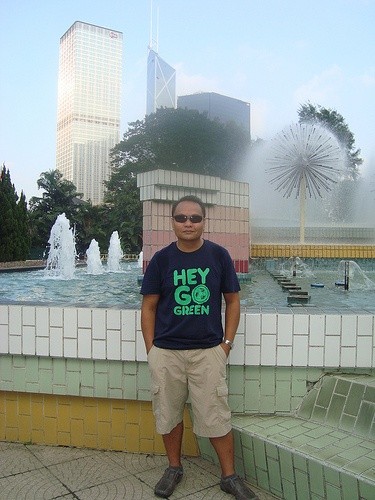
[173, 214, 202, 223]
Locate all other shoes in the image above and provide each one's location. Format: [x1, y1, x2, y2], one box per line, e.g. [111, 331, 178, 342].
[220, 475, 260, 500]
[154, 469, 184, 498]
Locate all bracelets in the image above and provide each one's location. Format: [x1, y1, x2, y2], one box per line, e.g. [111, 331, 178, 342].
[222, 339, 234, 349]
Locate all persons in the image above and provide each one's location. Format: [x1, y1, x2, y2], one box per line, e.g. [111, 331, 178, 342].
[137, 194, 260, 499]
